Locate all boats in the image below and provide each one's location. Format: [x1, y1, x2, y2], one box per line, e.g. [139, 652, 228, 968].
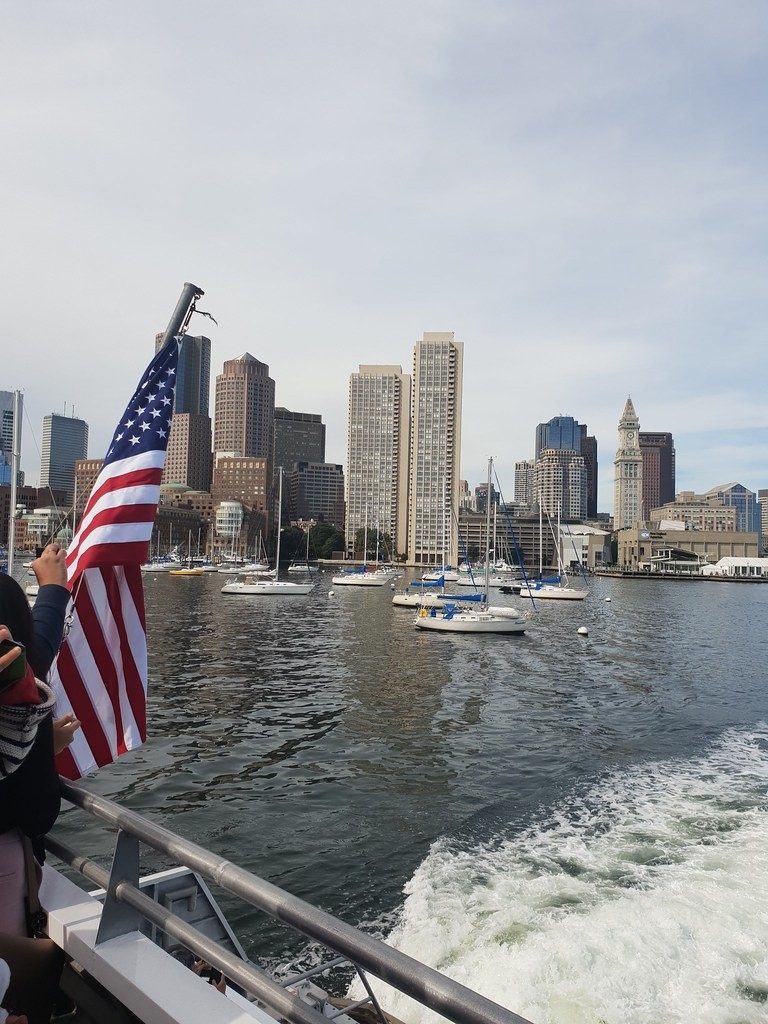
[460, 559, 512, 573]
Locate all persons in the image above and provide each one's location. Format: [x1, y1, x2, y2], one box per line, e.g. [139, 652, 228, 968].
[0, 542, 82, 1024]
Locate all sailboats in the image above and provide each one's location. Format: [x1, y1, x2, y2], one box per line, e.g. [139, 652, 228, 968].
[419, 475, 461, 582]
[499, 495, 591, 601]
[457, 501, 505, 587]
[140, 523, 276, 576]
[332, 502, 395, 587]
[364, 522, 405, 576]
[0, 473, 78, 596]
[415, 455, 540, 635]
[222, 466, 315, 595]
[287, 525, 320, 572]
[391, 496, 486, 609]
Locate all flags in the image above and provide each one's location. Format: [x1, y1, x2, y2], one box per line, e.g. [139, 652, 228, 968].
[45, 334, 182, 783]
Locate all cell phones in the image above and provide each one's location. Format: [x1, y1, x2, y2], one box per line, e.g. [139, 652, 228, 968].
[35, 547, 60, 559]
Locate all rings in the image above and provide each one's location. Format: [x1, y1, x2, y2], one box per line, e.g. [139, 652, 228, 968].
[47, 549, 57, 554]
